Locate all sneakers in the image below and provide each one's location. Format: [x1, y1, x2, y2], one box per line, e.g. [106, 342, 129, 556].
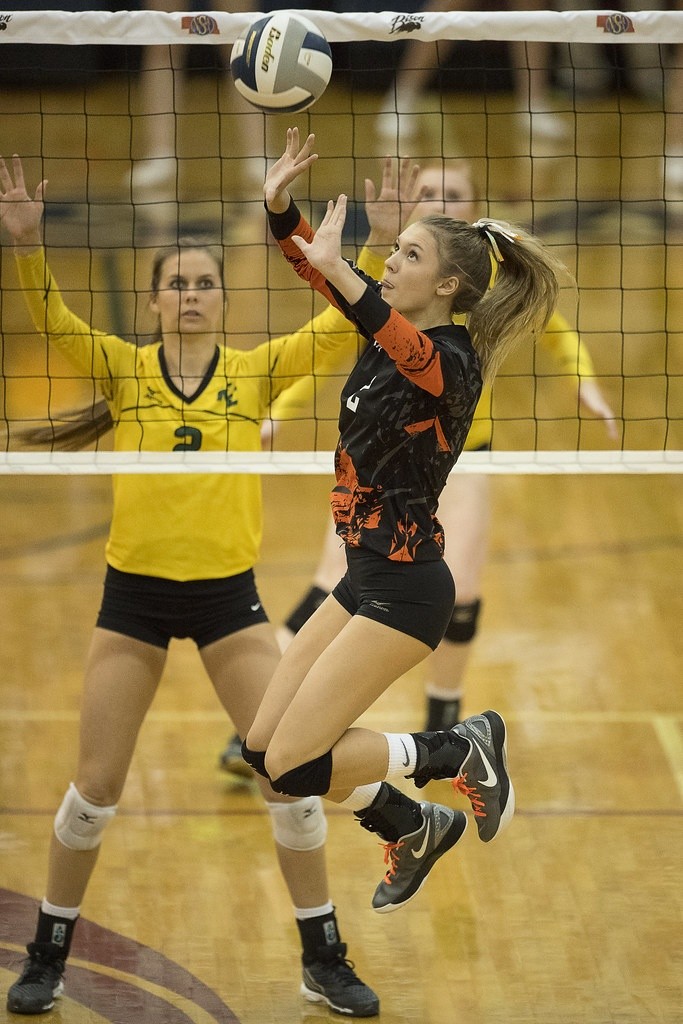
[372, 800, 469, 914]
[300, 942, 379, 1016]
[450, 710, 515, 843]
[7, 942, 66, 1013]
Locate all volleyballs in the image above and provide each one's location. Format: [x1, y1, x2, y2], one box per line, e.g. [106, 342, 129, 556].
[230, 14, 332, 113]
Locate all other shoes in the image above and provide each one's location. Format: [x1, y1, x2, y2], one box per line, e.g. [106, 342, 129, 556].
[220, 745, 253, 779]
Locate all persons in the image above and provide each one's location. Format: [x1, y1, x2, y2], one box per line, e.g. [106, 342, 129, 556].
[0, 153, 429, 1020]
[218, 158, 618, 780]
[119, 0, 683, 186]
[261, 127, 577, 915]
